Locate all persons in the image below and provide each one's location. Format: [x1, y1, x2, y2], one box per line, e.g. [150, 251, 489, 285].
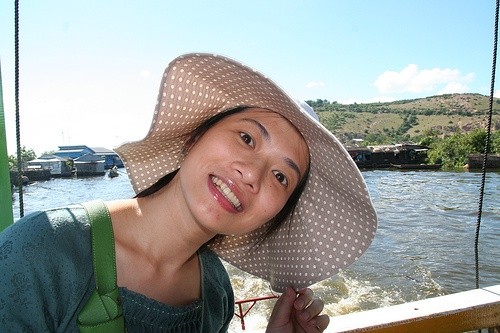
[109, 168, 114, 174]
[0, 49, 379, 333]
[113, 165, 118, 172]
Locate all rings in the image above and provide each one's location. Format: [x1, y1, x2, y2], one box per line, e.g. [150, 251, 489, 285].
[315, 324, 322, 332]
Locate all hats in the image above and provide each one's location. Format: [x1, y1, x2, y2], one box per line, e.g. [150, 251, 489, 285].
[112, 51, 380, 296]
